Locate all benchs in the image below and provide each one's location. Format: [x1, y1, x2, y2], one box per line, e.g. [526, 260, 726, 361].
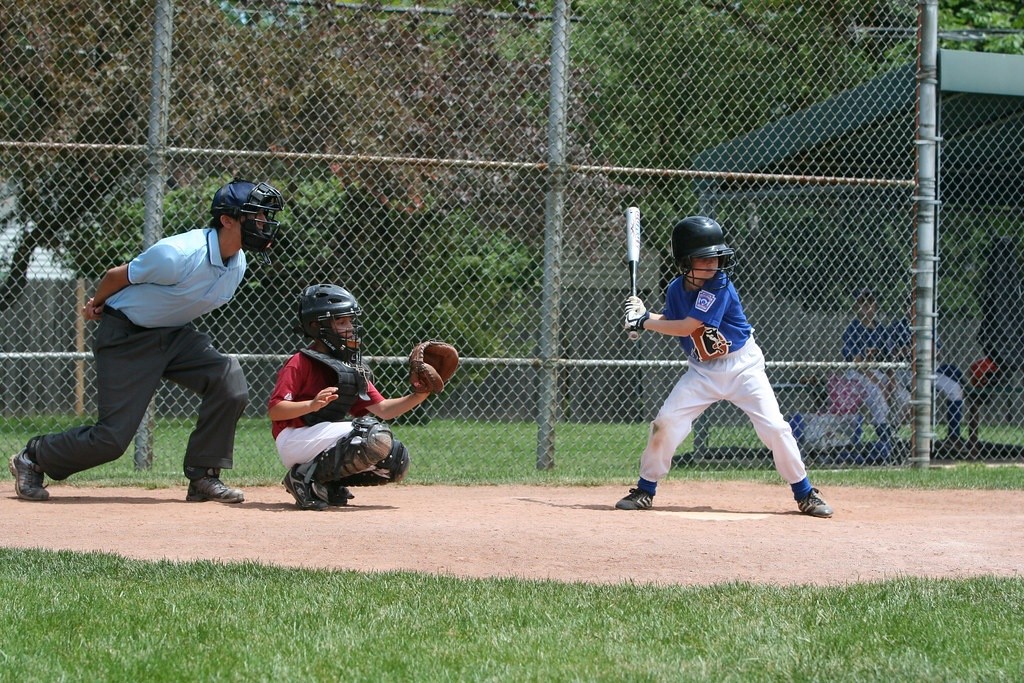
[693, 382, 992, 461]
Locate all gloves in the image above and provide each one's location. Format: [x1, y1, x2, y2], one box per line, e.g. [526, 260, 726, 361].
[625, 296, 649, 319]
[624, 311, 648, 330]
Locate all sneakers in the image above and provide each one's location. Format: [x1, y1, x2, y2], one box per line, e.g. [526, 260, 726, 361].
[186, 477, 244, 502]
[322, 481, 348, 505]
[281, 463, 328, 510]
[9, 448, 49, 501]
[798, 488, 834, 518]
[615, 487, 653, 510]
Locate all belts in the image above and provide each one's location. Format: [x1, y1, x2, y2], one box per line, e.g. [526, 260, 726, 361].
[102, 304, 128, 320]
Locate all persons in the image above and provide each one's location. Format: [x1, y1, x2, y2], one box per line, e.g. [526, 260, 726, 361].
[614, 216, 834, 520]
[841, 290, 990, 462]
[269, 283, 459, 510]
[9, 179, 285, 503]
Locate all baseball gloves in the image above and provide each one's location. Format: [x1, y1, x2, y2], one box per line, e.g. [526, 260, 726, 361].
[408, 336, 461, 394]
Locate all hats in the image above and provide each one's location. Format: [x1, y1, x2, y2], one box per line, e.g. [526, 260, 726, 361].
[210, 179, 279, 215]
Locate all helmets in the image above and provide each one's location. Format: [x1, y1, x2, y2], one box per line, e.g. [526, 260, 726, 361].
[298, 284, 362, 366]
[672, 216, 735, 270]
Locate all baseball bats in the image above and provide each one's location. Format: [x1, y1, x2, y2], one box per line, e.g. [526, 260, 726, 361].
[626, 207, 641, 341]
